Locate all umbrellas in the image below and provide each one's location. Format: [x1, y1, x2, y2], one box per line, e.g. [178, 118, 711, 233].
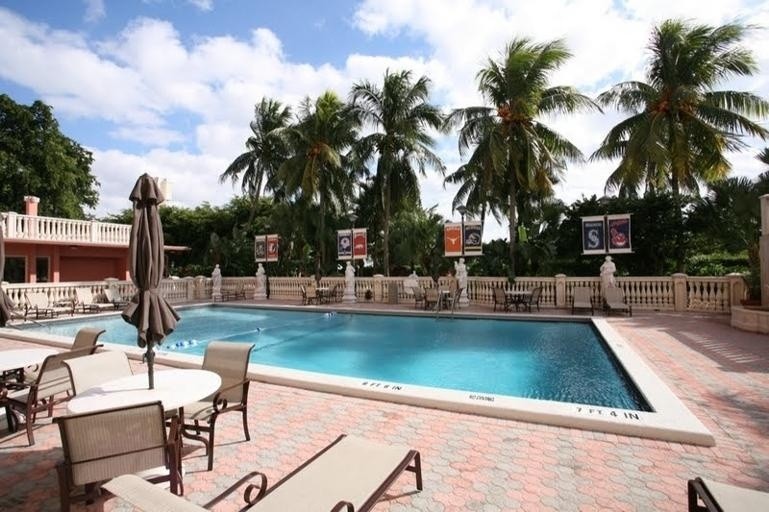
[432, 248, 440, 292]
[1, 212, 14, 327]
[315, 257, 322, 287]
[121, 172, 181, 388]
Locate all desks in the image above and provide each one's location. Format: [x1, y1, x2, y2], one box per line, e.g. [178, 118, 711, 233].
[2, 348, 59, 428]
[68, 366, 223, 469]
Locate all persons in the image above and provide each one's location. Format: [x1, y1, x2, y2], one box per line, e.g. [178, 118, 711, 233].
[345, 261, 356, 291]
[212, 264, 222, 292]
[600, 255, 616, 289]
[256, 262, 265, 289]
[454, 258, 467, 294]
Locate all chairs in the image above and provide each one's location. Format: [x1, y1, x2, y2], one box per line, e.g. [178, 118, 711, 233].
[299, 280, 544, 313]
[6, 341, 105, 446]
[100, 433, 422, 512]
[48, 397, 187, 508]
[49, 330, 106, 416]
[168, 338, 256, 470]
[59, 349, 136, 396]
[24, 287, 129, 321]
